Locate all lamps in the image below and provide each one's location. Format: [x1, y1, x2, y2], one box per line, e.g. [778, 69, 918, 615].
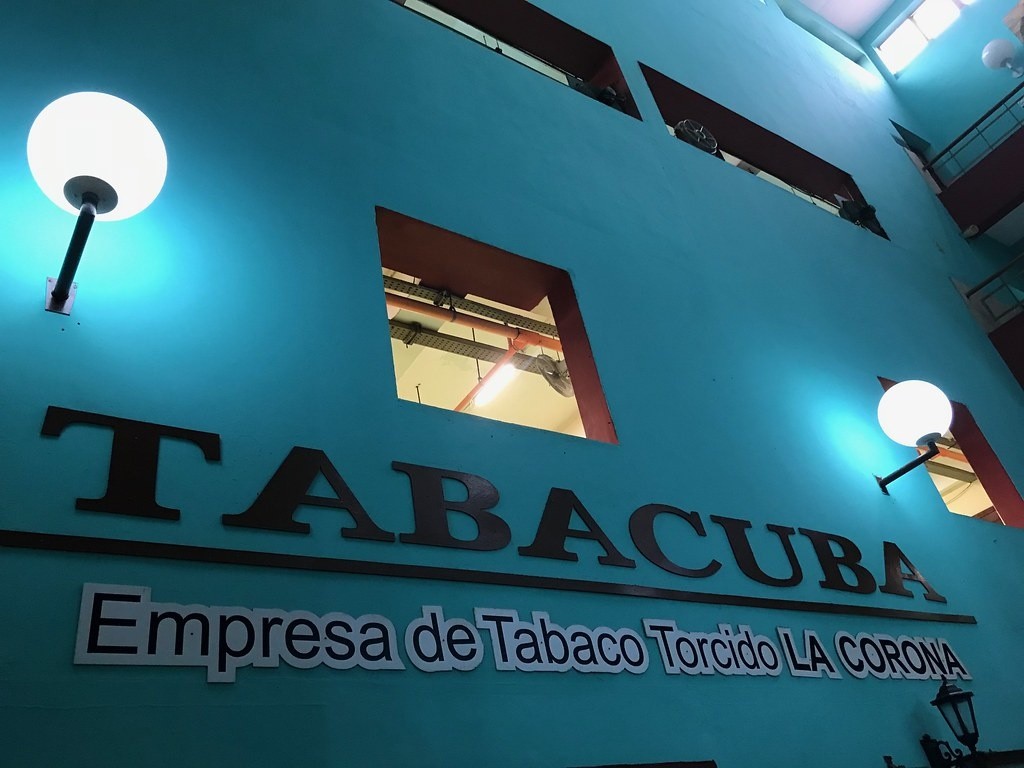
[872, 379, 955, 496]
[982, 38, 1024, 78]
[27, 90, 170, 315]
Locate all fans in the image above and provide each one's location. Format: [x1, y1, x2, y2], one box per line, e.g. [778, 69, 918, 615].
[536, 354, 574, 398]
[675, 119, 718, 154]
